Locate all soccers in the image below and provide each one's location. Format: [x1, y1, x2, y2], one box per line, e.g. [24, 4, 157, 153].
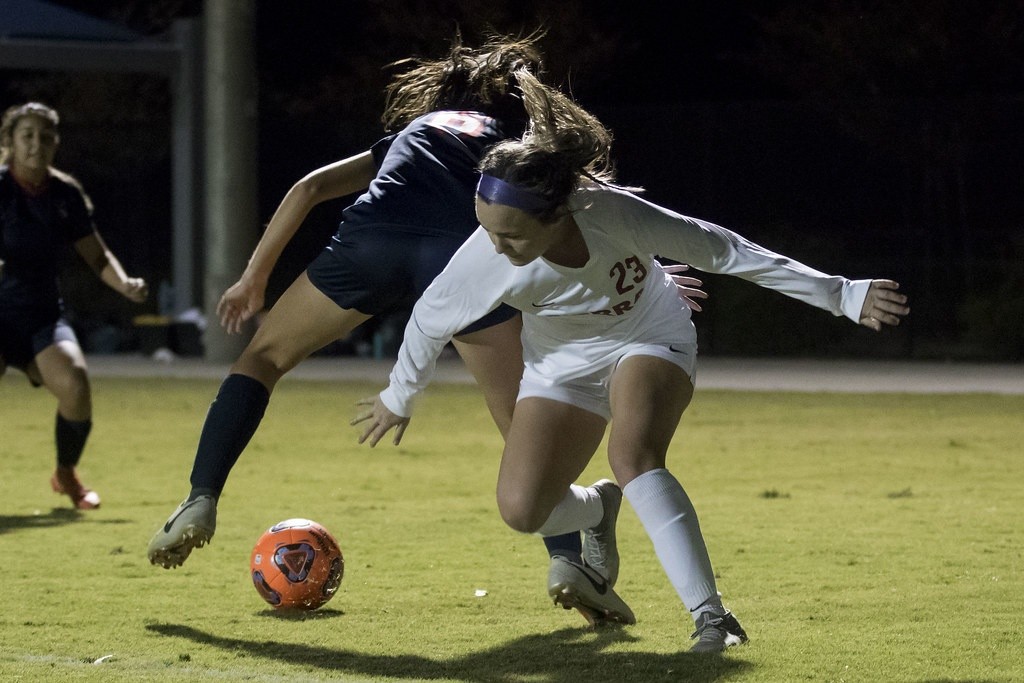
[248, 516, 345, 613]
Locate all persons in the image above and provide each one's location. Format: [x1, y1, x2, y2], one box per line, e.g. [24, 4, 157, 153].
[349, 124, 910, 656]
[0, 101, 150, 507]
[146, 28, 710, 632]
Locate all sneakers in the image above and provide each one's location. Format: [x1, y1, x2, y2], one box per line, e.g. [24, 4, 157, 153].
[690, 609, 750, 653]
[547, 555, 636, 625]
[582, 479, 622, 588]
[147, 495, 216, 569]
[51, 468, 100, 509]
